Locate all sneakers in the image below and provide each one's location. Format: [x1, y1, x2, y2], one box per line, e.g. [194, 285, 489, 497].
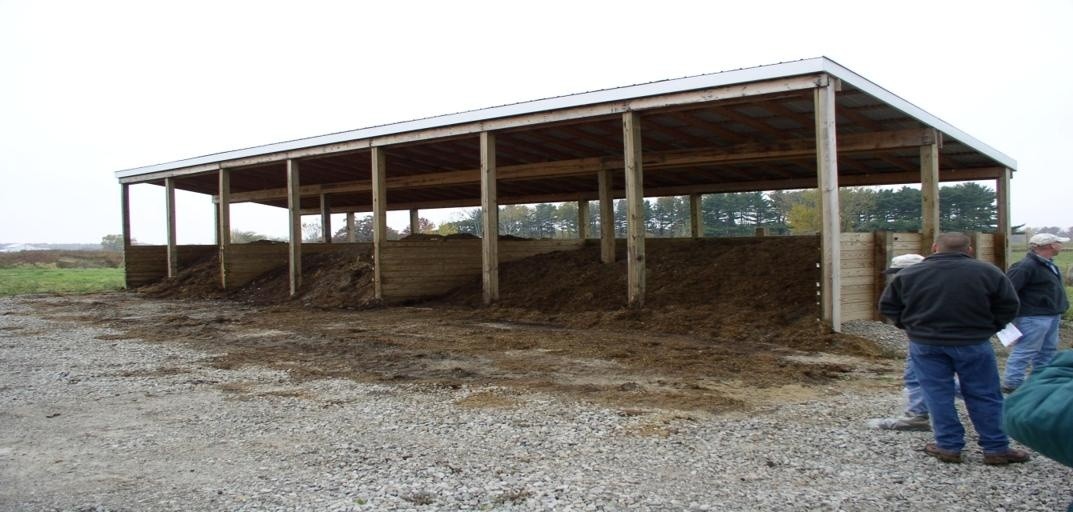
[1000, 385, 1012, 393]
[923, 441, 962, 464]
[981, 446, 1030, 466]
[877, 410, 931, 433]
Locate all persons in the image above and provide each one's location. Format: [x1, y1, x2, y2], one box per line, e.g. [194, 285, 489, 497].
[875, 226, 1032, 466]
[1001, 233, 1071, 396]
[872, 254, 932, 432]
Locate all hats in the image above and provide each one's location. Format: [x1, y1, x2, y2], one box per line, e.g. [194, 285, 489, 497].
[1026, 231, 1072, 250]
[879, 251, 925, 277]
